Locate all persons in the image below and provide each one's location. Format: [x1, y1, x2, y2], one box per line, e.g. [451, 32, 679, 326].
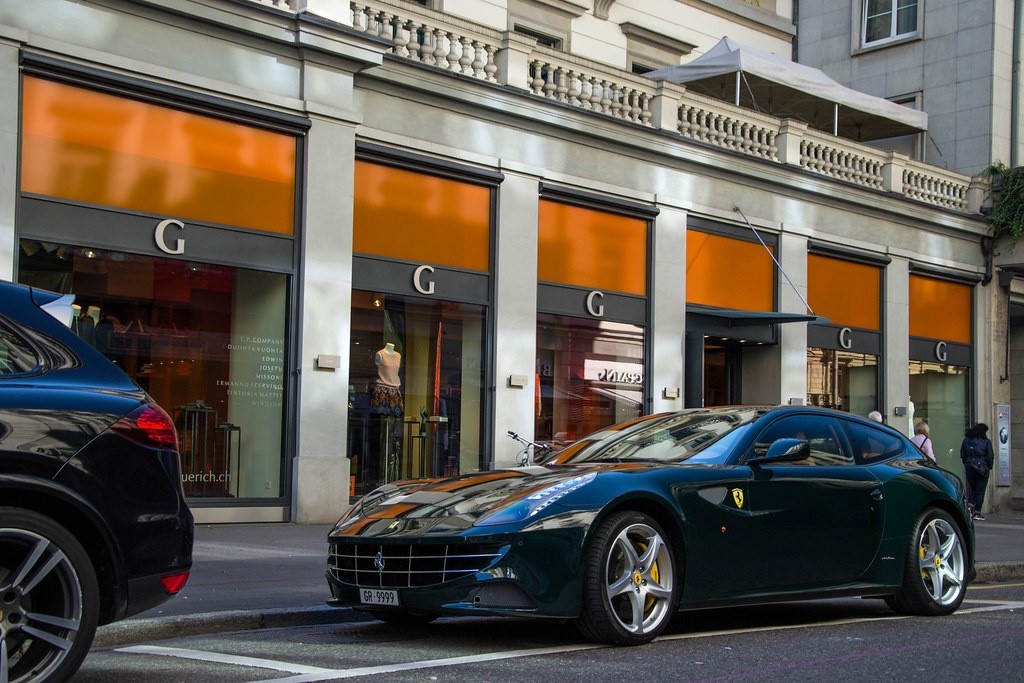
[373, 343, 405, 418]
[911, 422, 936, 463]
[960, 423, 994, 520]
[566, 364, 593, 425]
[860, 411, 900, 458]
[68, 304, 114, 359]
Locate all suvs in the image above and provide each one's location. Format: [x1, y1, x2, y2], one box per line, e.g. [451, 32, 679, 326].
[0, 278, 196, 682]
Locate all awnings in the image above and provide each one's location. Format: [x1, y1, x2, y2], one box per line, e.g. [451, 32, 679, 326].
[686, 309, 820, 322]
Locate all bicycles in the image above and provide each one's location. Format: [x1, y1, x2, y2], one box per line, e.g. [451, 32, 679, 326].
[507, 430, 550, 466]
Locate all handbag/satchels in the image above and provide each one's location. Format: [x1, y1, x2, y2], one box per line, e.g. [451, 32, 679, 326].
[969, 439, 989, 476]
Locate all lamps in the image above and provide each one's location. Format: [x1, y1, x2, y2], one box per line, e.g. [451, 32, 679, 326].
[81, 248, 101, 259]
[370, 292, 384, 309]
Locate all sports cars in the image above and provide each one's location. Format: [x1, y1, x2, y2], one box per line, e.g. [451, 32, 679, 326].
[322, 405, 978, 647]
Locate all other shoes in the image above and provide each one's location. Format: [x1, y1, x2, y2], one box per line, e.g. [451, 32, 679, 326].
[968, 506, 973, 518]
[972, 513, 985, 521]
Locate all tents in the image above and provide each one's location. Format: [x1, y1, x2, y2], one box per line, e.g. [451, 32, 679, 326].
[639, 34, 928, 163]
